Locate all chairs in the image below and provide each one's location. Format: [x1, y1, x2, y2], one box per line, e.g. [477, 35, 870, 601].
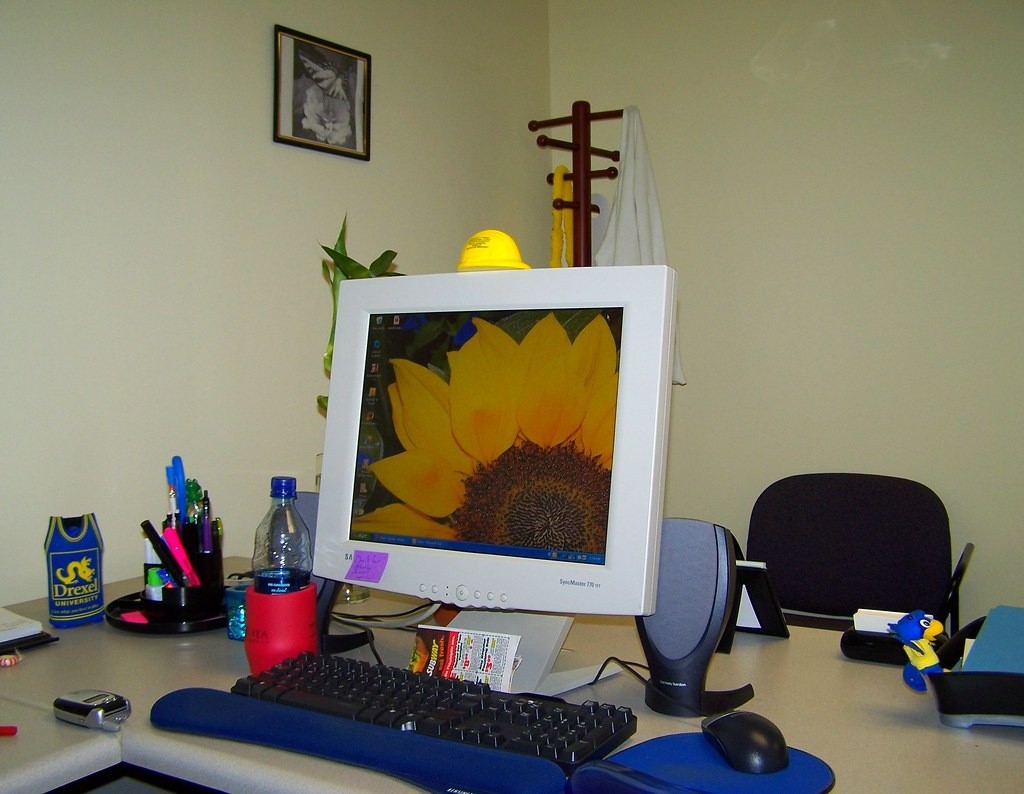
[743, 472, 975, 641]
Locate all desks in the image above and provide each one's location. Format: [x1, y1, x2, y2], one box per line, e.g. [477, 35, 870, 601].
[0, 553, 1024, 794]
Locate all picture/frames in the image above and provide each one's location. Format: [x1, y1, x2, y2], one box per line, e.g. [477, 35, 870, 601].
[272, 21, 372, 161]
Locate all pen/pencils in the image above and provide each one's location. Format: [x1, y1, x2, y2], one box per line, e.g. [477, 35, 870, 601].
[134, 453, 222, 603]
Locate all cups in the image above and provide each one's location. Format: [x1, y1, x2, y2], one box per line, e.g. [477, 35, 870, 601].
[242, 582, 322, 678]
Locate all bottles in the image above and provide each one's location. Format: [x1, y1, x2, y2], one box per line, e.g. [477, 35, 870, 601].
[251, 475, 309, 593]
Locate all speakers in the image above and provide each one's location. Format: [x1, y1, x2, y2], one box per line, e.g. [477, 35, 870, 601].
[635, 518, 754, 715]
[295, 491, 374, 652]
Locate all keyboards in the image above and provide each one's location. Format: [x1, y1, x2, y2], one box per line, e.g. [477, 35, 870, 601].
[230, 649, 637, 776]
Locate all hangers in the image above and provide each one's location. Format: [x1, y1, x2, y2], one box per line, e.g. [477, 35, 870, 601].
[529, 100, 640, 267]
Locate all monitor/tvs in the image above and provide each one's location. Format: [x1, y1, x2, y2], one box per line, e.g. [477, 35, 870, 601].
[312, 264, 680, 617]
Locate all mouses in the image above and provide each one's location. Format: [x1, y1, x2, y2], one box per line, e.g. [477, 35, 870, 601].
[701, 709, 789, 774]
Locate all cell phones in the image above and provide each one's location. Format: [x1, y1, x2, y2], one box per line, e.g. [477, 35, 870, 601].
[53, 689, 132, 733]
